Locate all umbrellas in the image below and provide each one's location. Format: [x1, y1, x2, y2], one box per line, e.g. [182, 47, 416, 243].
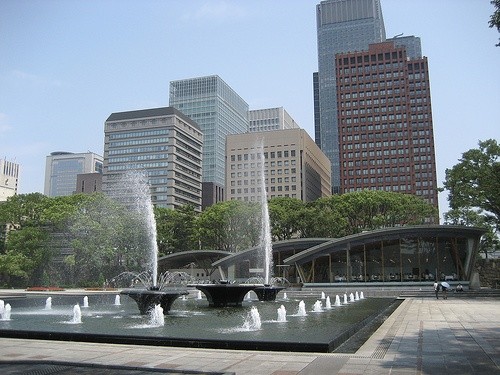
[441, 282, 450, 287]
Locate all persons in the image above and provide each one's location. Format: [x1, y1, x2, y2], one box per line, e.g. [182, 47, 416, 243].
[334, 272, 459, 281]
[433, 281, 439, 300]
[454, 283, 464, 293]
[442, 285, 448, 300]
